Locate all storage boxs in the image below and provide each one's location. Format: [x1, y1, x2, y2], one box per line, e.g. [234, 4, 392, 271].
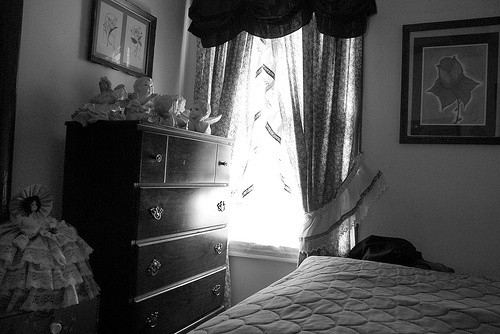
[0, 294, 99, 334]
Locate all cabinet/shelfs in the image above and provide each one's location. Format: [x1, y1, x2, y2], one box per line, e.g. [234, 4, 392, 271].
[62, 121, 226, 334]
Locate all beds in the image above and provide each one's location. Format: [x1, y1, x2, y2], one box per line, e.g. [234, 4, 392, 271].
[187, 256, 500, 334]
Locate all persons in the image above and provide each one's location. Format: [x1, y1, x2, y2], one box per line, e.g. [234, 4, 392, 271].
[0, 184, 101, 311]
[71, 76, 223, 135]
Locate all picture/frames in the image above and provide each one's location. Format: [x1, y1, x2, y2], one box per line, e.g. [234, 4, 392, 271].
[399, 16, 500, 145]
[87, 0, 157, 79]
